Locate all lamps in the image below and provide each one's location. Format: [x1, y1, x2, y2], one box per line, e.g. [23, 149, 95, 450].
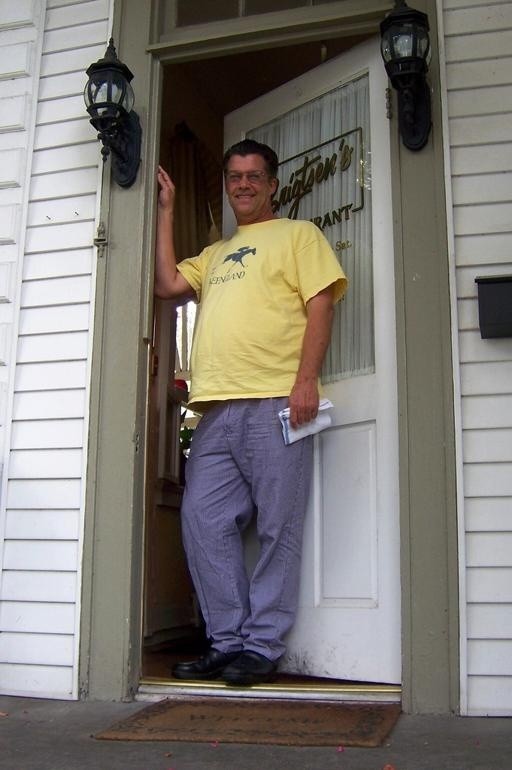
[82, 36, 142, 189]
[379, 1, 433, 152]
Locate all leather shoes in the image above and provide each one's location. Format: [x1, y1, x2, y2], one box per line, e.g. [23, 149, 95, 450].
[172, 647, 237, 680]
[222, 651, 276, 687]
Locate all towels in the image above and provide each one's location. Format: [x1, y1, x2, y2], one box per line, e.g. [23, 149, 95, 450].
[278, 399, 334, 446]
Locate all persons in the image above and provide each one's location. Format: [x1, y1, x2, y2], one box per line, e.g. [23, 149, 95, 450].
[154, 139, 349, 686]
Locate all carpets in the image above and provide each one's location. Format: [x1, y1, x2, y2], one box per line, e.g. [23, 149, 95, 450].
[95, 696, 403, 748]
